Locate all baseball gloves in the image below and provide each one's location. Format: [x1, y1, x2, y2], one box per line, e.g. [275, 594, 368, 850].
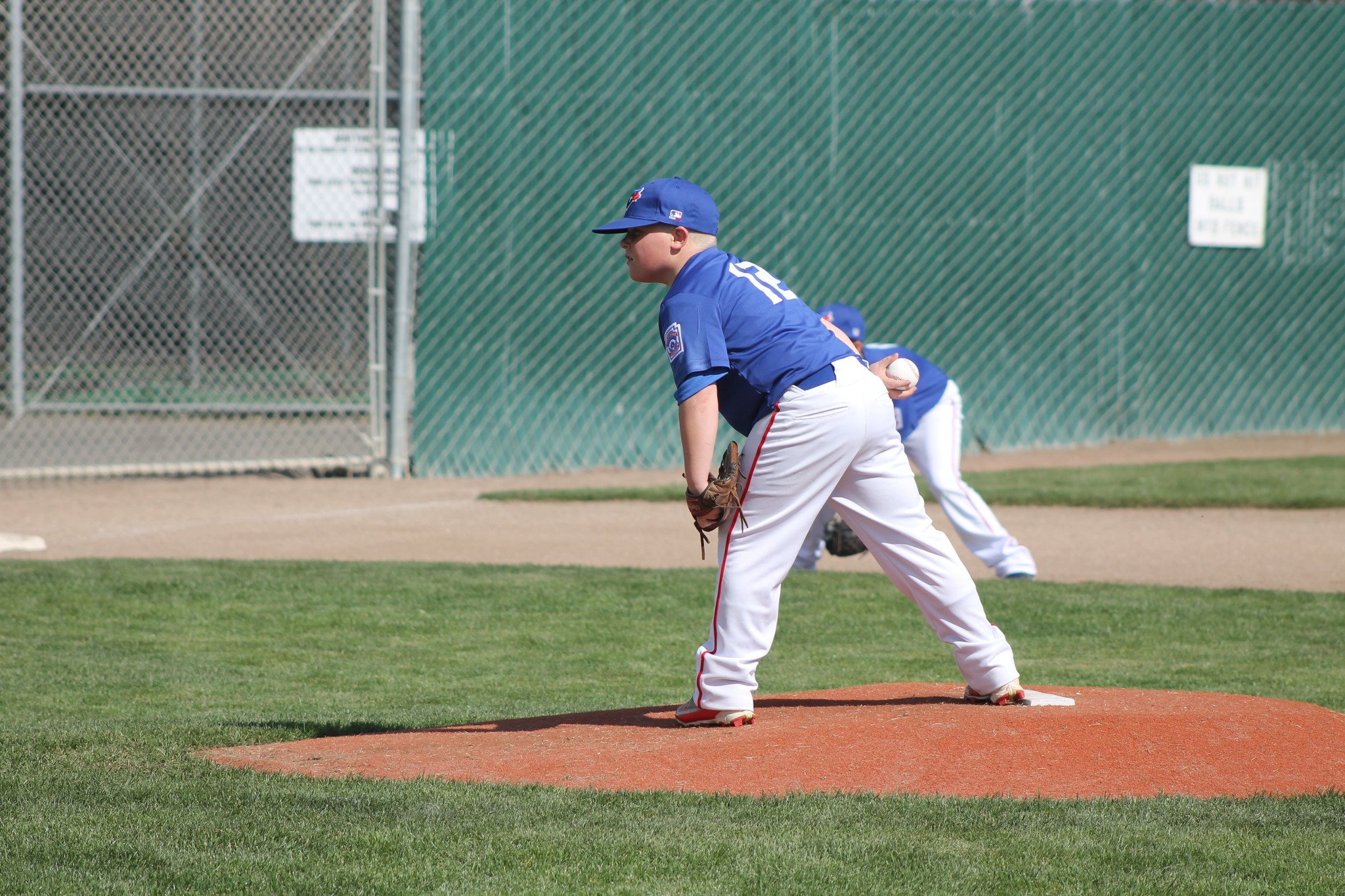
[686, 443, 742, 534]
[823, 520, 868, 556]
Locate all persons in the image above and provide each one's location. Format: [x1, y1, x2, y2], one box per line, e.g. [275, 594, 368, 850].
[793, 306, 1036, 580]
[590, 176, 1024, 726]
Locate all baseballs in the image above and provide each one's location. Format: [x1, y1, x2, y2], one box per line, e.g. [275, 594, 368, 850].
[886, 357, 920, 391]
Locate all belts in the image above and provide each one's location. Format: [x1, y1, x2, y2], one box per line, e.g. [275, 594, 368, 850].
[798, 356, 865, 389]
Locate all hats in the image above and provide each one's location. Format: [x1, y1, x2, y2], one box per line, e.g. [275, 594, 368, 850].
[593, 175, 718, 236]
[817, 306, 864, 340]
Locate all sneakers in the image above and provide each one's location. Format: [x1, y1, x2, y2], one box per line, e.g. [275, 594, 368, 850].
[674, 693, 756, 729]
[965, 675, 1025, 705]
